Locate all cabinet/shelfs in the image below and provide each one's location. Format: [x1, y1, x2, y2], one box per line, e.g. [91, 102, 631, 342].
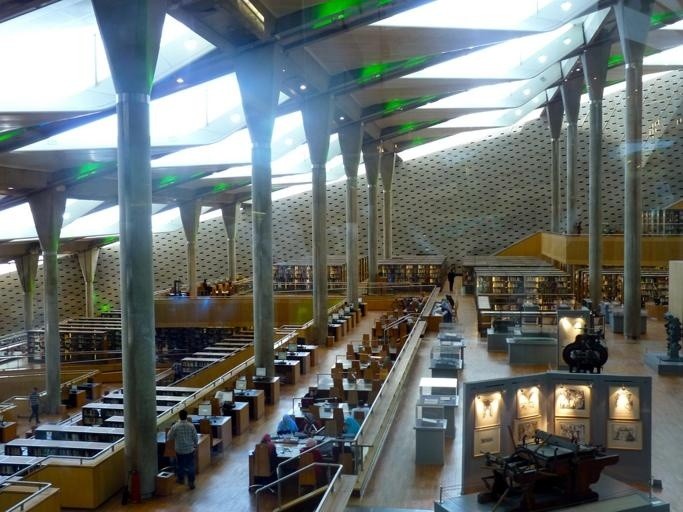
[27, 330, 109, 365]
[477, 275, 668, 309]
[0, 393, 124, 512]
[273, 265, 442, 295]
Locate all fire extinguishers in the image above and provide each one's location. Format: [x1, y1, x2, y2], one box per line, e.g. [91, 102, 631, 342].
[121, 468, 141, 505]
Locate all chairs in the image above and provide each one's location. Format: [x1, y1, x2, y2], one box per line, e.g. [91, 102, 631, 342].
[213, 283, 234, 296]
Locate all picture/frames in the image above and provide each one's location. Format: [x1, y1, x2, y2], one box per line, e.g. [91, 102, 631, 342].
[472, 383, 644, 458]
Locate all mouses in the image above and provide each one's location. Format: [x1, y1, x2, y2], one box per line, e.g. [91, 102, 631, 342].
[289, 363, 291, 364]
[297, 353, 298, 355]
[214, 420, 216, 423]
[247, 393, 249, 395]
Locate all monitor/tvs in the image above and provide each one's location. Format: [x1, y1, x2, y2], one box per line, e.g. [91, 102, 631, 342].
[235, 379, 247, 395]
[288, 343, 297, 355]
[45, 431, 52, 441]
[255, 367, 267, 377]
[277, 351, 287, 364]
[358, 297, 363, 303]
[327, 302, 354, 324]
[21, 445, 29, 457]
[222, 390, 234, 404]
[297, 336, 305, 348]
[319, 404, 335, 420]
[198, 404, 212, 419]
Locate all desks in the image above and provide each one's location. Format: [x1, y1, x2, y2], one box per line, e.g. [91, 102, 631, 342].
[62, 382, 102, 408]
[157, 388, 265, 492]
[246, 304, 418, 491]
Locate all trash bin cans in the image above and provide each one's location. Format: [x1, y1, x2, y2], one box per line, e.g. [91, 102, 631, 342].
[327, 335, 335, 347]
[155, 472, 178, 497]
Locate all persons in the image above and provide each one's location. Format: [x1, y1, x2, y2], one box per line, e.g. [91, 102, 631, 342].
[254, 414, 360, 479]
[167, 410, 198, 489]
[399, 269, 456, 321]
[203, 279, 210, 289]
[29, 387, 41, 424]
